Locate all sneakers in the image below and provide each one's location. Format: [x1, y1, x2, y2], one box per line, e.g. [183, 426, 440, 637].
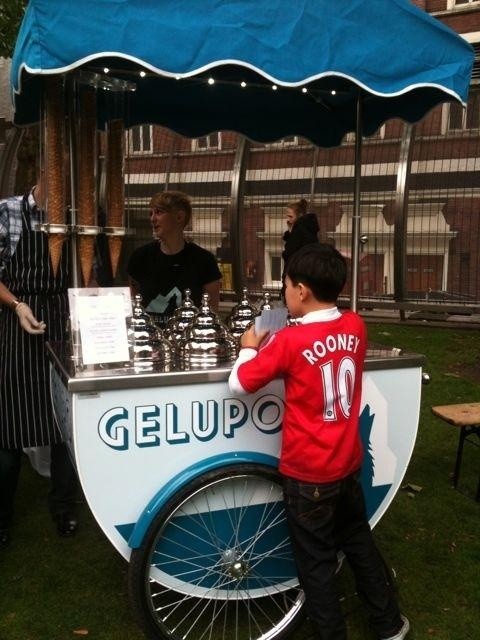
[384, 613, 409, 640]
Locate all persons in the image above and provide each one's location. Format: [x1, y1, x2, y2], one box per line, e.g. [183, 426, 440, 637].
[226, 243, 410, 639]
[0, 170, 116, 545]
[282, 197, 321, 298]
[123, 190, 225, 328]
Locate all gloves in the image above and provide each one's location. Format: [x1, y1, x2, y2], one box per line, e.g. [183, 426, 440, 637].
[15, 303, 46, 335]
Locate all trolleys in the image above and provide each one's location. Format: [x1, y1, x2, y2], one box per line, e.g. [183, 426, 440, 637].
[40, 334, 429, 640]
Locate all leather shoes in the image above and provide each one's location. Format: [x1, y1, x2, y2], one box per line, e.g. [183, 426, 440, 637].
[0, 531, 10, 551]
[56, 511, 78, 536]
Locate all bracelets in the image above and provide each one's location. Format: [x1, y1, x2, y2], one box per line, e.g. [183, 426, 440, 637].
[10, 299, 22, 311]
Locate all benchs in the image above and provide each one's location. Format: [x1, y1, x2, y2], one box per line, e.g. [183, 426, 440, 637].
[430, 402, 480, 490]
